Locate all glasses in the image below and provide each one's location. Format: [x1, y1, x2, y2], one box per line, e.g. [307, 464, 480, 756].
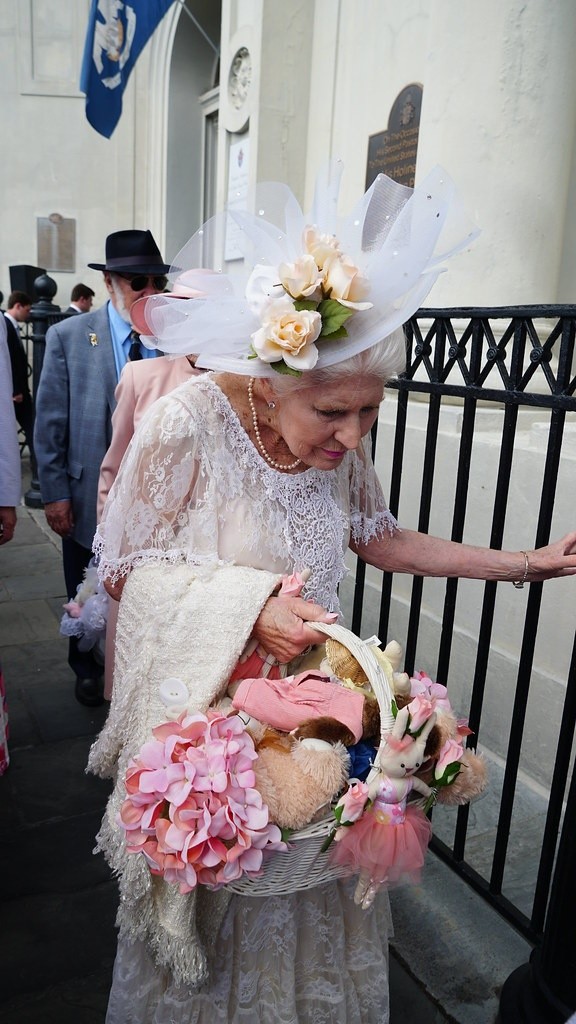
[114, 271, 168, 291]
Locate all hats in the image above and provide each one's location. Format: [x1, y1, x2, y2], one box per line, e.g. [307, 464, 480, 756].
[87, 229, 181, 273]
[140, 163, 480, 378]
[130, 268, 254, 352]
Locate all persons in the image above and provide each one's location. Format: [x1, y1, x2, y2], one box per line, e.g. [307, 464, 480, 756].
[65, 283, 95, 314]
[0, 229, 216, 708]
[64, 314, 576, 1024]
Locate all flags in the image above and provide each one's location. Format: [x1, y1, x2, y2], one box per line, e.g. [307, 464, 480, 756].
[79, 0, 174, 139]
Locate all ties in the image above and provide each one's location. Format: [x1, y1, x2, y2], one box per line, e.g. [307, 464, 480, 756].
[128, 333, 144, 361]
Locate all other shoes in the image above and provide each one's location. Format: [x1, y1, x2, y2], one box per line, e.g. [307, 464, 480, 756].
[74, 670, 105, 707]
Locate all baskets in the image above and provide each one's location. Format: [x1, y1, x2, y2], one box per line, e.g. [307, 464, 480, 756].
[206, 620, 442, 896]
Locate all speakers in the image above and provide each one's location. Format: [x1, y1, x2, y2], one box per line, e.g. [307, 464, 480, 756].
[9, 264, 48, 305]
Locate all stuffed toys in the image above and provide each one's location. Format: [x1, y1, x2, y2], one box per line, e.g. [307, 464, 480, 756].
[210, 640, 488, 830]
[77, 593, 108, 654]
[332, 707, 435, 911]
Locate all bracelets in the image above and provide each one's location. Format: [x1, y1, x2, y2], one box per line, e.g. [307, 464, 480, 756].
[513, 551, 529, 589]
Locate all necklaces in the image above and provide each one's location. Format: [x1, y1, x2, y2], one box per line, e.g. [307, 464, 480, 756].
[247, 378, 302, 470]
